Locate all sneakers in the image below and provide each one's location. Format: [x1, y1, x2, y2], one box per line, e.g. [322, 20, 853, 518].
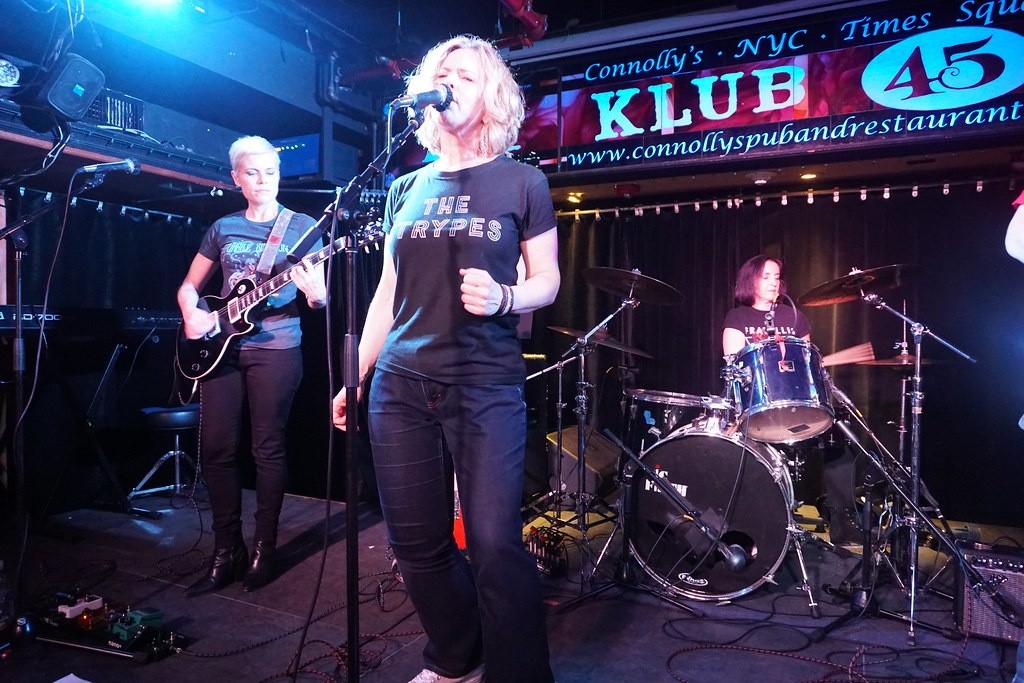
[408, 664, 483, 683]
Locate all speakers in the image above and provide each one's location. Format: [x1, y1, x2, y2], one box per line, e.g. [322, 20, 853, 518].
[544, 424, 626, 511]
[954, 535, 1024, 646]
[34, 52, 106, 121]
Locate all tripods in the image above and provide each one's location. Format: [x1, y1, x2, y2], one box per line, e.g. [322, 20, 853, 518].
[0, 172, 150, 666]
[521, 281, 962, 646]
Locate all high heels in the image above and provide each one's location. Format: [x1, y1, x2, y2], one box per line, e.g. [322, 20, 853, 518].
[239, 537, 278, 593]
[182, 541, 249, 597]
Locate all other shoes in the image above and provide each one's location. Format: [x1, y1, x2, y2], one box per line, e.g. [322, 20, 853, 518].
[829, 508, 884, 546]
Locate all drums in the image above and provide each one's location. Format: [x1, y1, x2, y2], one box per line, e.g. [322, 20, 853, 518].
[718, 335, 836, 444]
[614, 387, 739, 488]
[617, 410, 795, 601]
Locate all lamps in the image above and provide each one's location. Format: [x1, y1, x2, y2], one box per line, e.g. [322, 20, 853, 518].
[0, 55, 22, 87]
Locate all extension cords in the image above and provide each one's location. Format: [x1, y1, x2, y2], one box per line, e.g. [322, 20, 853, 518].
[59, 595, 103, 619]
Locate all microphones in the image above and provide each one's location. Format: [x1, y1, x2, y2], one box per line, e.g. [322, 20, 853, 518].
[389, 83, 453, 112]
[1001, 608, 1024, 629]
[76, 157, 142, 175]
[769, 296, 778, 321]
[720, 544, 749, 573]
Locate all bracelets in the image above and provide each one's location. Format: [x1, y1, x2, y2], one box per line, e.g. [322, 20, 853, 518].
[493, 284, 514, 316]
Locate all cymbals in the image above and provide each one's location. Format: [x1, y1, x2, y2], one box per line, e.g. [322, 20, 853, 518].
[581, 266, 686, 306]
[796, 262, 921, 307]
[852, 353, 937, 377]
[544, 324, 657, 360]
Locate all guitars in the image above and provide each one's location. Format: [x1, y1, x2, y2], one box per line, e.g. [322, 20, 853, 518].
[175, 219, 385, 385]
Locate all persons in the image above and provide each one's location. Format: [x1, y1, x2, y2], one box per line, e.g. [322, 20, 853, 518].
[178, 136, 326, 598]
[723, 255, 883, 545]
[333, 36, 561, 683]
